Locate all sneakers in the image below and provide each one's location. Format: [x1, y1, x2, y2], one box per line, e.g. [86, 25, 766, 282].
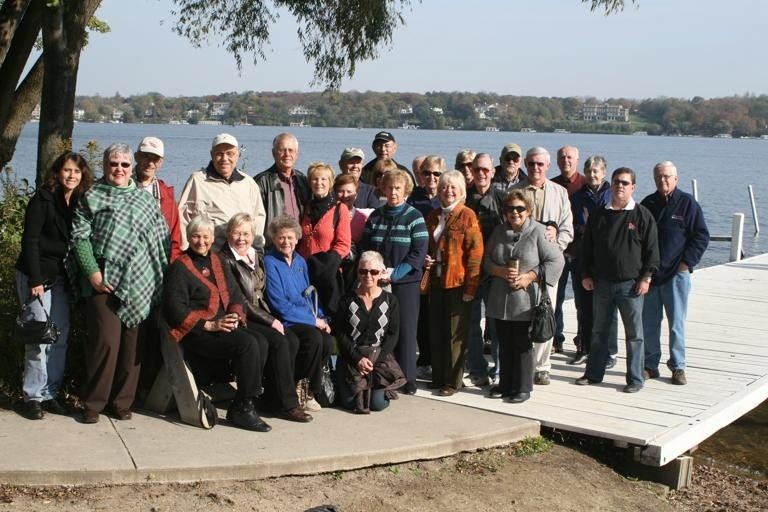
[534, 371, 550, 385]
[439, 386, 456, 396]
[571, 352, 587, 364]
[75, 409, 98, 423]
[606, 355, 617, 369]
[462, 374, 492, 387]
[553, 342, 563, 353]
[417, 365, 432, 380]
[104, 404, 131, 419]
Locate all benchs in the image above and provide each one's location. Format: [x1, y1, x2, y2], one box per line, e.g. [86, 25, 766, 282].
[145, 306, 265, 428]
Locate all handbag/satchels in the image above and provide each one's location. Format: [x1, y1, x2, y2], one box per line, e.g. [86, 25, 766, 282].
[11, 313, 60, 343]
[531, 291, 556, 343]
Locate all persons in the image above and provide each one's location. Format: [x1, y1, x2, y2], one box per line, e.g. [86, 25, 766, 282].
[12, 150, 97, 421]
[421, 169, 486, 397]
[576, 167, 661, 393]
[358, 168, 430, 397]
[640, 159, 710, 385]
[132, 132, 619, 413]
[213, 213, 314, 424]
[163, 215, 273, 432]
[482, 190, 566, 402]
[334, 251, 401, 413]
[62, 141, 172, 425]
[257, 214, 335, 411]
[177, 133, 267, 254]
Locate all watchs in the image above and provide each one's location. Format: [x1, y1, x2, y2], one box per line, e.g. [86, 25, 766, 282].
[642, 277, 651, 283]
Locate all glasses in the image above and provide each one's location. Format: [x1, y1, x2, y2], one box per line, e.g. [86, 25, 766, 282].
[109, 162, 131, 167]
[613, 179, 629, 186]
[422, 171, 442, 176]
[504, 156, 519, 162]
[506, 207, 526, 213]
[455, 162, 473, 168]
[527, 162, 544, 167]
[375, 144, 391, 148]
[359, 269, 379, 275]
[472, 168, 490, 173]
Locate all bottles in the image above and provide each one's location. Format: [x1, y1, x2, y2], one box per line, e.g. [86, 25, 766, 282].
[508, 257, 519, 288]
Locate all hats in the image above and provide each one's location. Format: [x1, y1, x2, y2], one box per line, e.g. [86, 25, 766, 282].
[137, 137, 164, 157]
[502, 143, 521, 157]
[341, 147, 364, 160]
[212, 134, 238, 147]
[372, 132, 395, 141]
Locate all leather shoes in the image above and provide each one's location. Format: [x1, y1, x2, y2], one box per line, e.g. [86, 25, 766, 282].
[623, 383, 643, 392]
[41, 399, 65, 414]
[488, 387, 505, 398]
[576, 377, 592, 385]
[644, 368, 659, 379]
[278, 407, 313, 422]
[24, 400, 43, 419]
[506, 391, 530, 402]
[227, 401, 272, 431]
[403, 380, 416, 395]
[671, 369, 687, 384]
